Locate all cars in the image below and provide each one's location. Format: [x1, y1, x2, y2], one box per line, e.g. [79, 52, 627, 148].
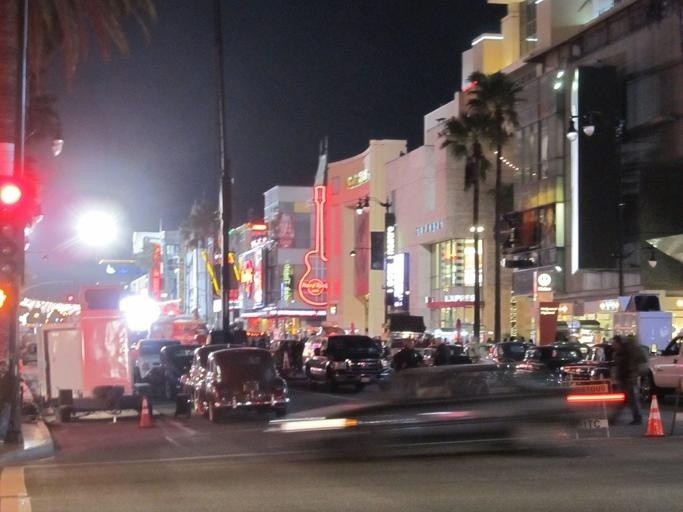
[265, 362, 626, 460]
[513, 345, 583, 381]
[154, 343, 199, 400]
[375, 338, 533, 380]
[193, 347, 290, 422]
[264, 340, 302, 378]
[177, 343, 248, 421]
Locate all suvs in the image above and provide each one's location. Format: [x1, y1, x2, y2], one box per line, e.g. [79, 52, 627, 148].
[22, 333, 38, 365]
[556, 343, 649, 401]
[638, 327, 683, 406]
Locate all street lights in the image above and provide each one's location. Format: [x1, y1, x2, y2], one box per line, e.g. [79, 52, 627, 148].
[565, 109, 627, 296]
[352, 196, 390, 337]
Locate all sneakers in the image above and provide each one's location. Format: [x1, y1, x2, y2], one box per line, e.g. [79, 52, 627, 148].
[628, 419, 642, 426]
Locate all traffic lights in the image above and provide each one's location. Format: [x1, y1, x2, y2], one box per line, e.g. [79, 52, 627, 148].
[0, 237, 20, 310]
[0, 176, 33, 230]
[32, 308, 41, 322]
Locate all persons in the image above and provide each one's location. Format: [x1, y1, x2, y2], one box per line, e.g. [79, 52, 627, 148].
[259, 323, 304, 342]
[603, 330, 647, 425]
[488, 337, 533, 343]
[381, 329, 449, 347]
[278, 213, 295, 247]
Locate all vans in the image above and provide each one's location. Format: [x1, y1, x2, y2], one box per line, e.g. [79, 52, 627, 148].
[132, 339, 179, 385]
[147, 316, 207, 348]
[300, 334, 383, 393]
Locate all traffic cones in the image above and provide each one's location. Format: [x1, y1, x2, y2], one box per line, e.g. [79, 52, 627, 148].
[137, 397, 156, 429]
[642, 395, 666, 437]
